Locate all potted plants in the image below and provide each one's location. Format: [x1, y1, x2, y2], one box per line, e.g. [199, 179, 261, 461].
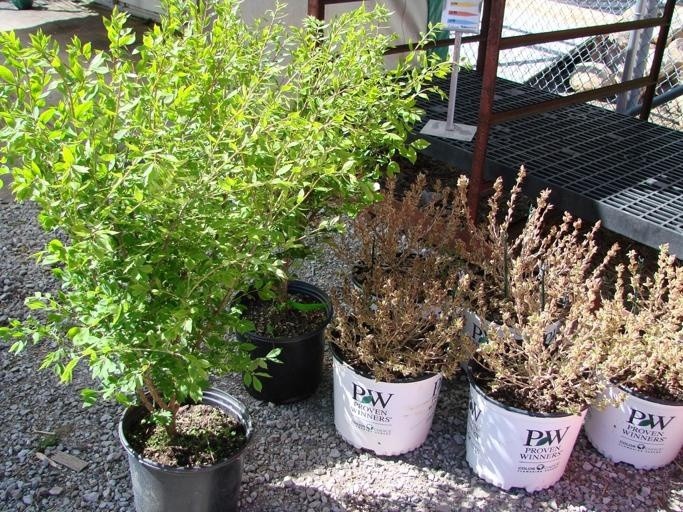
[471, 168, 576, 344]
[464, 247, 608, 493]
[583, 243, 682, 472]
[325, 254, 468, 456]
[230, 28, 473, 407]
[340, 176, 461, 310]
[3, 28, 355, 512]
